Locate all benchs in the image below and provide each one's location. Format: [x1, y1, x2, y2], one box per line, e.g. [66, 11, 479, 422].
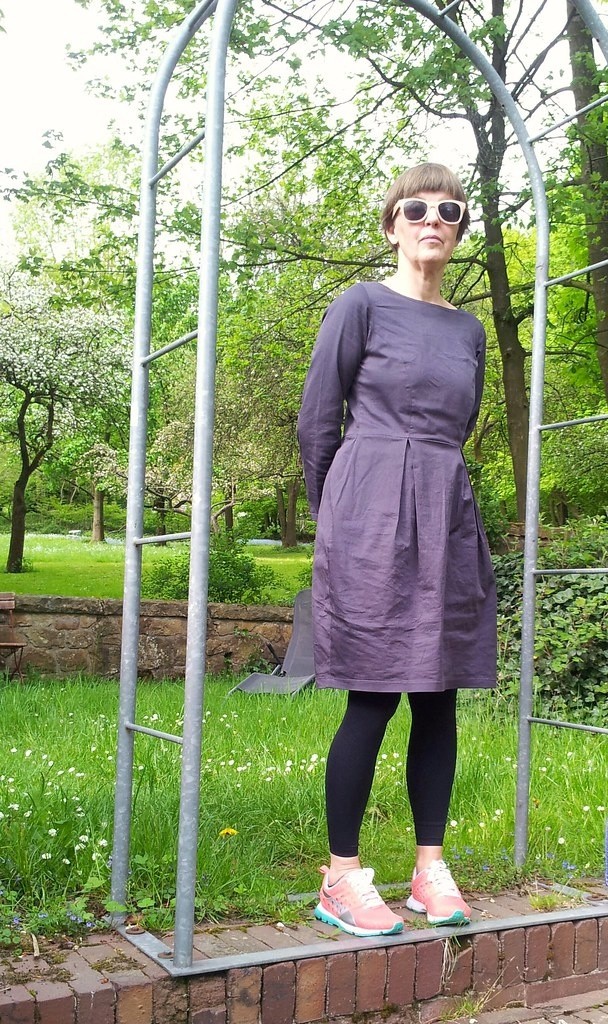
[0, 593, 32, 686]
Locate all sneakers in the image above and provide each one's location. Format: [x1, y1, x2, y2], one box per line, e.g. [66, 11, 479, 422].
[405, 860, 471, 925]
[313, 865, 404, 936]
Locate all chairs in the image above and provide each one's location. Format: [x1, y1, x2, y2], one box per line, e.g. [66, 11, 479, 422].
[227, 590, 315, 698]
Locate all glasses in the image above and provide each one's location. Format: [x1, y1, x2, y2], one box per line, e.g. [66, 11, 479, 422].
[392, 198, 467, 225]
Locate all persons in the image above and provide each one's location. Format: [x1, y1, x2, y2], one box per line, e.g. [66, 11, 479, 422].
[296, 162, 499, 937]
